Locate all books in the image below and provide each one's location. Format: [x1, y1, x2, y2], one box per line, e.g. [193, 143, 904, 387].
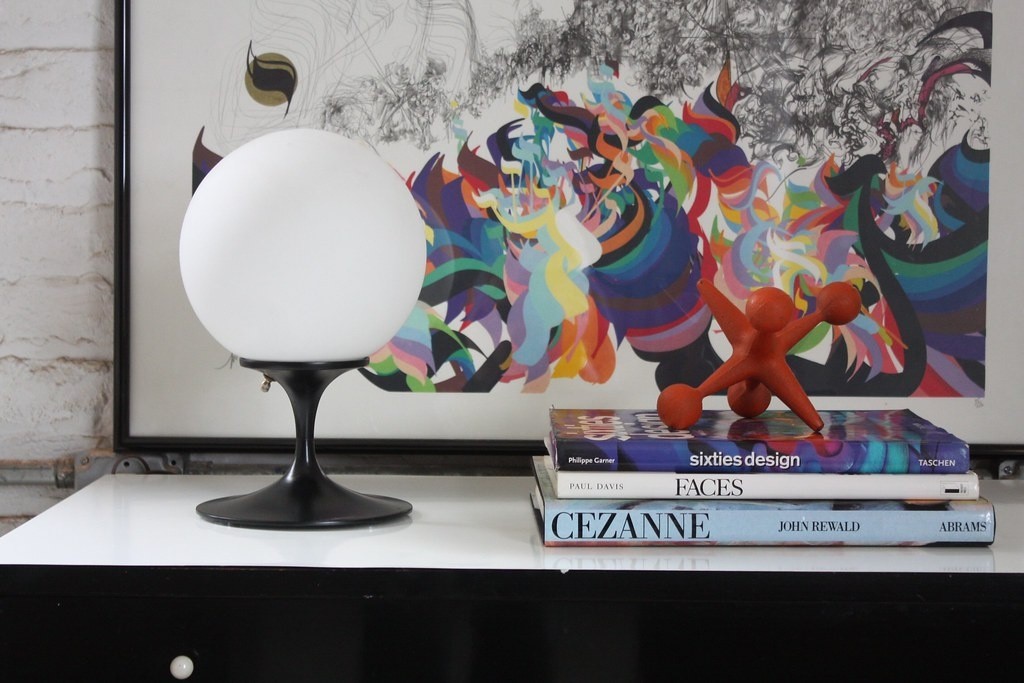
[541, 408, 981, 500]
[528, 454, 994, 548]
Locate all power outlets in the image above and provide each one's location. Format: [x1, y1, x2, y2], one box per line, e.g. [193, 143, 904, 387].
[74, 449, 185, 491]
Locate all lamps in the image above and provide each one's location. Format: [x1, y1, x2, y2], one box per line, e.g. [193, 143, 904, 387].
[178, 128, 426, 532]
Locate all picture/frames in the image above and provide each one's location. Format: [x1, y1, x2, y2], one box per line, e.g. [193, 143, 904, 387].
[112, 0, 1024, 467]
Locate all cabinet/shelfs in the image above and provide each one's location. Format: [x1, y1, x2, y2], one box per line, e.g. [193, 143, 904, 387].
[0, 473, 1024, 683]
